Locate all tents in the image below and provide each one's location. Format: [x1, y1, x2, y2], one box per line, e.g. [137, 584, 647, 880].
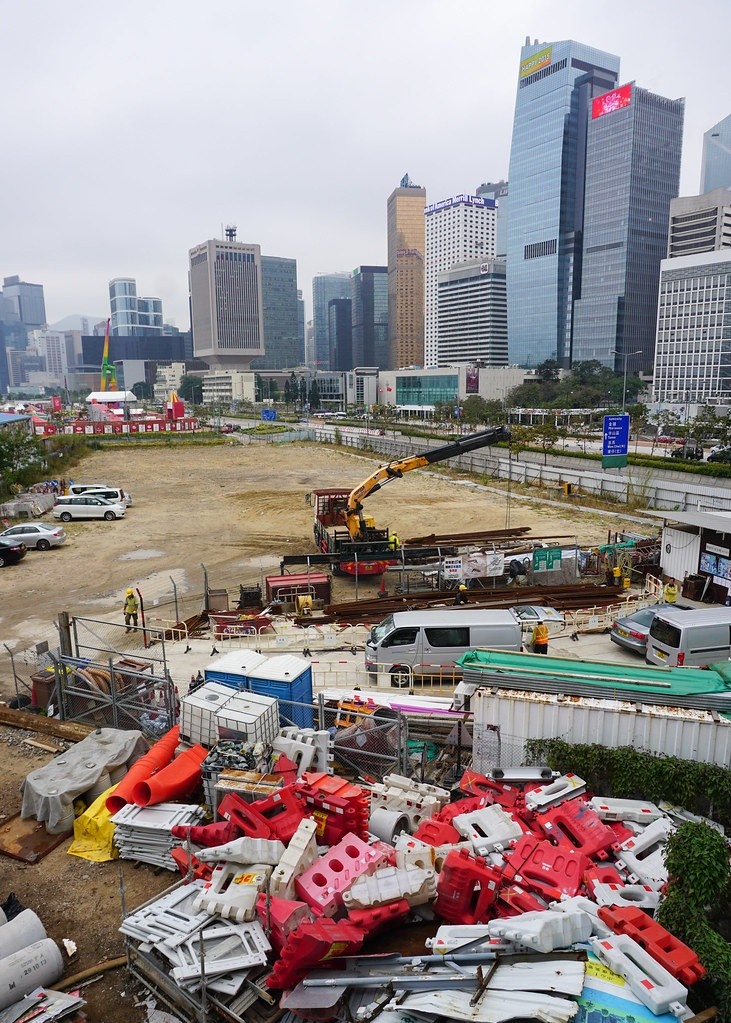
[0, 402, 49, 427]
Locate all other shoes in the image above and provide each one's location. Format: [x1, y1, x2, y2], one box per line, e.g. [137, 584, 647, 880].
[126, 629, 130, 633]
[133, 630, 137, 632]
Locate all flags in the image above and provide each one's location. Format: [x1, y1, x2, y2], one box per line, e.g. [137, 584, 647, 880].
[53, 397, 61, 411]
[378, 388, 383, 392]
[387, 387, 391, 391]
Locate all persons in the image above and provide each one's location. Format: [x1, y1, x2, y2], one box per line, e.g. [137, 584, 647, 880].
[527, 621, 549, 654]
[664, 577, 678, 603]
[68, 477, 74, 489]
[123, 589, 139, 634]
[389, 530, 402, 550]
[53, 486, 58, 495]
[45, 487, 50, 493]
[452, 585, 472, 606]
[60, 479, 65, 496]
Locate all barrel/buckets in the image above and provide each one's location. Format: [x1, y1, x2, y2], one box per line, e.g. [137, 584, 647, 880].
[624, 578, 631, 588]
[612, 567, 621, 577]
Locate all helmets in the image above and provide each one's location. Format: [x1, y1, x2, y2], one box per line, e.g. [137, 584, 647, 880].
[392, 531, 397, 535]
[459, 585, 468, 591]
[126, 589, 133, 596]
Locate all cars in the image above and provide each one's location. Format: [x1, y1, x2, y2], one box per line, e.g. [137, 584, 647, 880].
[0, 522, 67, 551]
[710, 445, 730, 453]
[0, 536, 28, 568]
[707, 449, 731, 464]
[609, 603, 696, 656]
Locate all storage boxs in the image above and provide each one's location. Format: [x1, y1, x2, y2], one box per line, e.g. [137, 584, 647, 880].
[474, 686, 731, 777]
[266, 572, 331, 604]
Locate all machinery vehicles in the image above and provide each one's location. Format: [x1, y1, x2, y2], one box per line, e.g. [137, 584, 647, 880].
[305, 422, 513, 576]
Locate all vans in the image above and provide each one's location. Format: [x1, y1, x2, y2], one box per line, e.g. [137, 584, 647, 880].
[653, 423, 687, 445]
[69, 484, 132, 508]
[645, 607, 731, 671]
[313, 412, 347, 420]
[364, 607, 565, 689]
[670, 445, 703, 461]
[80, 488, 127, 513]
[54, 495, 126, 522]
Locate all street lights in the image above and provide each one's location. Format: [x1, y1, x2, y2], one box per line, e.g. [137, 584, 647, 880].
[610, 349, 643, 416]
[566, 391, 574, 405]
[649, 384, 666, 427]
[496, 388, 504, 413]
